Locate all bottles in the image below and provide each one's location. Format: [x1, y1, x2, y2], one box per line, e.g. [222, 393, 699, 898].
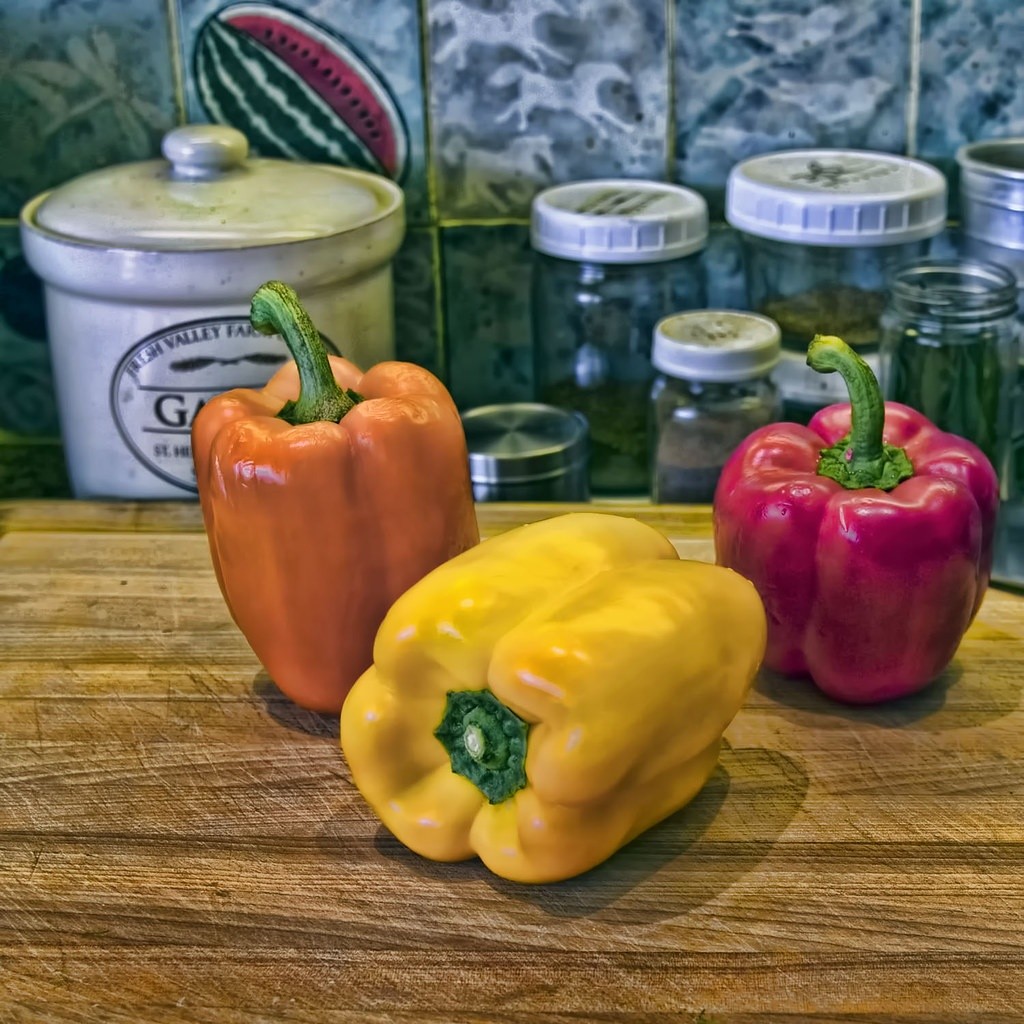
[455, 93, 1020, 551]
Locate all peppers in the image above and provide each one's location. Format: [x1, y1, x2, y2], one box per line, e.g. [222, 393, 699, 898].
[189, 284, 1000, 882]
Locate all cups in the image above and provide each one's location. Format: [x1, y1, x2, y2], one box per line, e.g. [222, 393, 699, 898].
[20, 129, 415, 502]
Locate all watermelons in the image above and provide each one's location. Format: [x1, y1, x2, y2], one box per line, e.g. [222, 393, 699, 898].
[193, 1, 411, 190]
